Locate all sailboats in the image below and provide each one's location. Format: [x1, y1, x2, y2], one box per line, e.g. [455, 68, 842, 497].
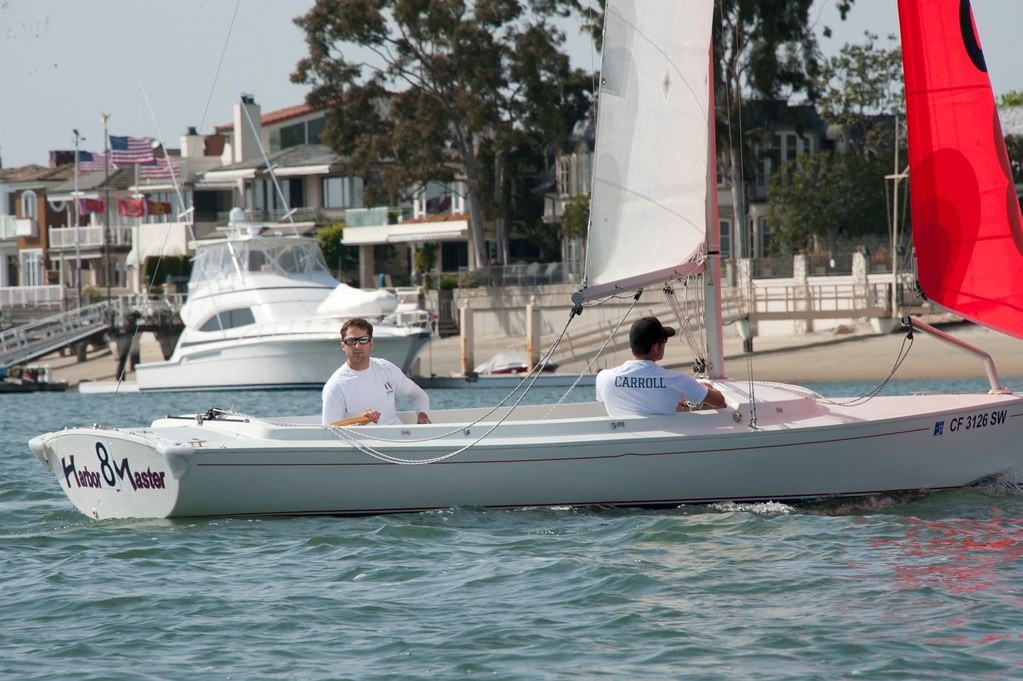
[26, 0, 1023, 521]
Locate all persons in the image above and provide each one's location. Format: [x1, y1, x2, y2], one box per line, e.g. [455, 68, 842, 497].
[489, 256, 503, 286]
[596, 317, 725, 416]
[417, 288, 425, 310]
[322, 318, 432, 425]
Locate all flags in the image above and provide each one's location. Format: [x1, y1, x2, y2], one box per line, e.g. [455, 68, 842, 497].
[79, 153, 112, 171]
[110, 135, 155, 165]
[118, 198, 143, 217]
[140, 156, 180, 179]
[147, 200, 171, 214]
[78, 198, 103, 216]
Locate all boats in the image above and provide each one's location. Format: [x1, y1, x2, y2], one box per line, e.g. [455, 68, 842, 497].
[476, 351, 559, 376]
[79, 65, 436, 394]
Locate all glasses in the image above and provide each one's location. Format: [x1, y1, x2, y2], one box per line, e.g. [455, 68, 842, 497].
[344, 336, 372, 346]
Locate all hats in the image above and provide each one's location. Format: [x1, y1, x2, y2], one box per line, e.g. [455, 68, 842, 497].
[630, 317, 675, 347]
